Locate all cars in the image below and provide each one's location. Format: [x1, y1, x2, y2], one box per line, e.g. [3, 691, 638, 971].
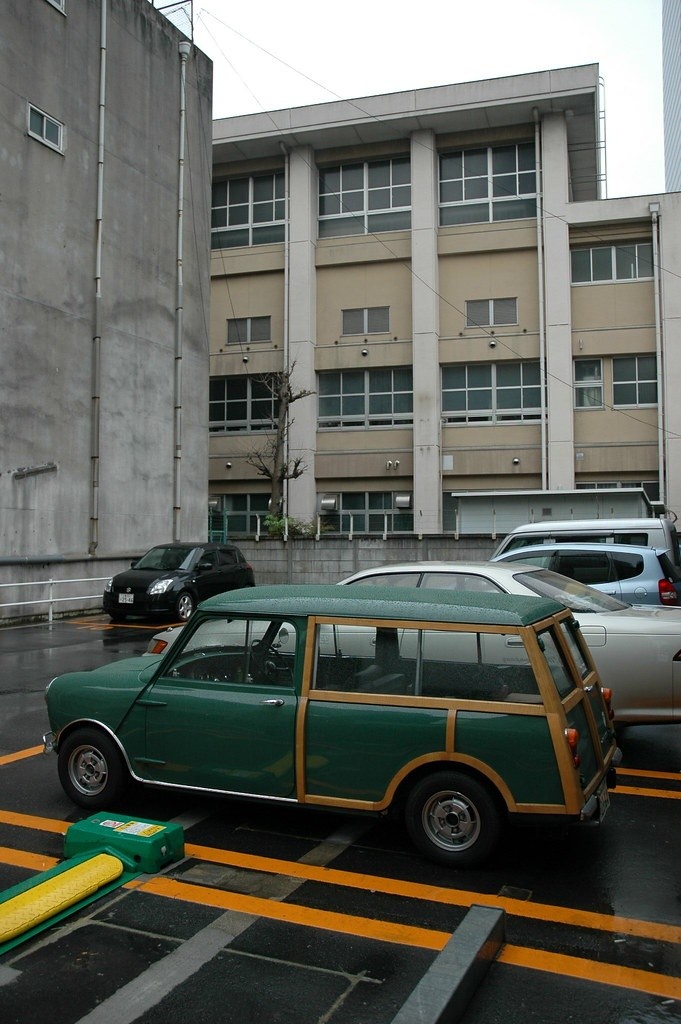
[140, 554, 681, 729]
[480, 541, 681, 606]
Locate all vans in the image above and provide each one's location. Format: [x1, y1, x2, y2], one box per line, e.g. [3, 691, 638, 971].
[40, 583, 626, 867]
[487, 517, 681, 569]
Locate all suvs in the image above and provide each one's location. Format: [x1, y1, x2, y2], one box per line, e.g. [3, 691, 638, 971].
[102, 542, 256, 624]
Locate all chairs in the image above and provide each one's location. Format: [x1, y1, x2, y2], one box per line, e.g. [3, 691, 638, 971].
[352, 665, 385, 688]
